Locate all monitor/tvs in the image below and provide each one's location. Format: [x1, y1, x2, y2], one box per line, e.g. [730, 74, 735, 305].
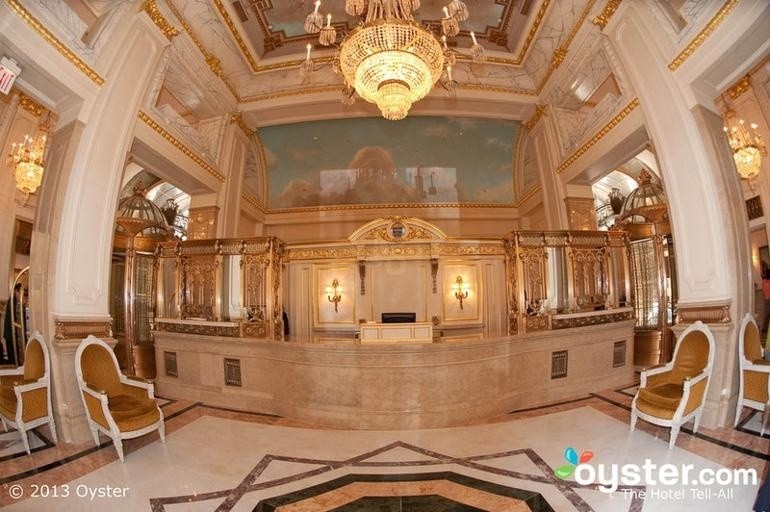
[382, 313, 416, 322]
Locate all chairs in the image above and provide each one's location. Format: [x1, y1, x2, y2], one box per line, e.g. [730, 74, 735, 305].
[74, 336, 166, 464]
[0, 332, 58, 456]
[733, 313, 770, 437]
[630, 321, 716, 448]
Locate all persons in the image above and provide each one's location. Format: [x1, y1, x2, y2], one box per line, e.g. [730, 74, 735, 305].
[2, 281, 21, 365]
[760, 261, 770, 361]
[282, 304, 289, 335]
[21, 286, 29, 341]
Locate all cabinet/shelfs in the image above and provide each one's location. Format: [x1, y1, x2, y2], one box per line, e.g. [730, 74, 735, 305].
[361, 322, 433, 344]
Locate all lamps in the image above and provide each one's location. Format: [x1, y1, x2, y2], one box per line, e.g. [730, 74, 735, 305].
[300, 0, 487, 121]
[327, 279, 343, 312]
[722, 108, 767, 195]
[454, 276, 469, 310]
[8, 134, 46, 205]
